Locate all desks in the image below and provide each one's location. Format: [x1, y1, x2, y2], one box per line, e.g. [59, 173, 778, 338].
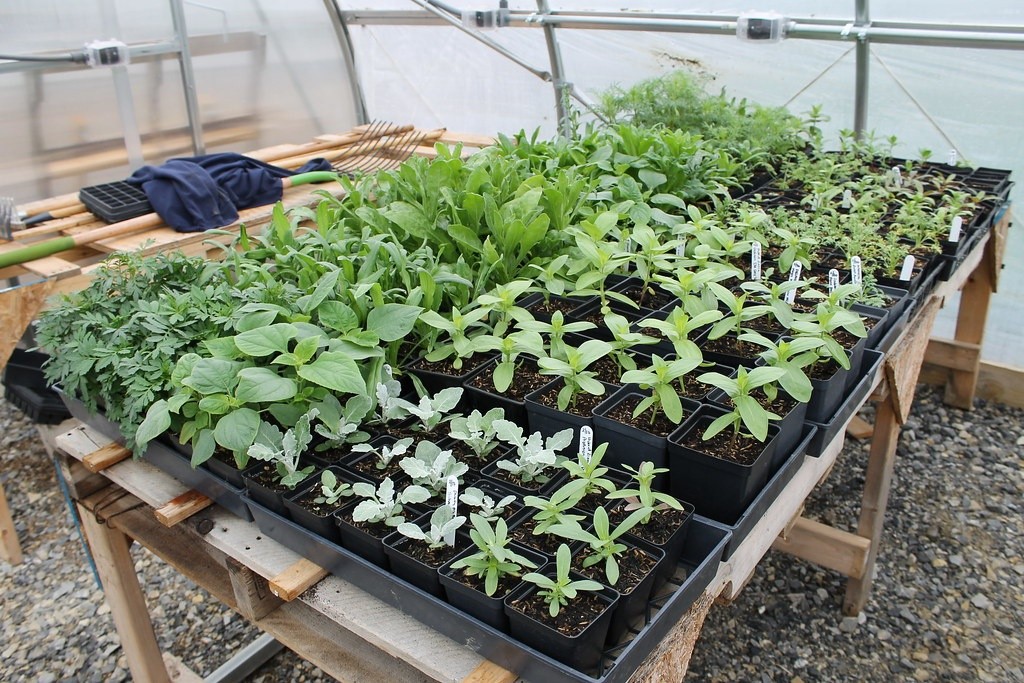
[53, 199, 1013, 683]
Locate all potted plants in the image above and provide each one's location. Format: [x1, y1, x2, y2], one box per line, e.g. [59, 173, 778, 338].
[333, 478, 432, 572]
[506, 488, 593, 562]
[26, 65, 1014, 519]
[569, 506, 665, 645]
[504, 544, 620, 672]
[605, 461, 695, 600]
[456, 479, 525, 536]
[436, 514, 548, 635]
[381, 505, 474, 601]
